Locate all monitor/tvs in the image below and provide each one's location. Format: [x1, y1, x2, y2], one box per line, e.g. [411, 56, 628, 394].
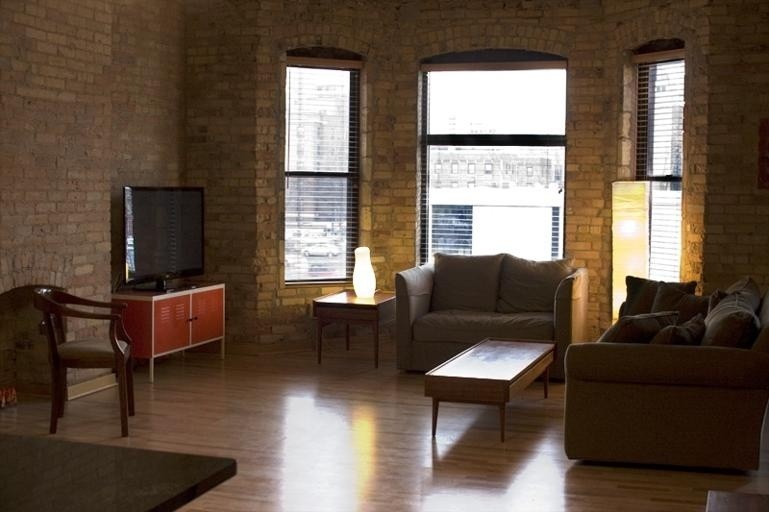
[122, 186, 206, 291]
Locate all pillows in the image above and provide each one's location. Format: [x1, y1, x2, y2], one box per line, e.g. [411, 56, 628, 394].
[597, 276, 740, 346]
[502, 254, 572, 315]
[430, 252, 501, 312]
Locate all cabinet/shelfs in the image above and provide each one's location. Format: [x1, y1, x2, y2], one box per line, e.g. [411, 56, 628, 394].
[109, 281, 227, 384]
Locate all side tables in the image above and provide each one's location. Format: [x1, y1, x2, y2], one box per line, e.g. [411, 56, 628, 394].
[312, 288, 396, 368]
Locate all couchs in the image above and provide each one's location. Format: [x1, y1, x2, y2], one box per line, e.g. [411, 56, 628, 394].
[563, 274, 769, 474]
[393, 248, 592, 384]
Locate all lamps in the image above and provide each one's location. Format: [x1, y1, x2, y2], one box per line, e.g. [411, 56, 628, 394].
[351, 246, 382, 299]
[611, 179, 650, 329]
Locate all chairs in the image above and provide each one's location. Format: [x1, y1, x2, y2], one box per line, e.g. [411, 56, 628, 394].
[31, 286, 136, 439]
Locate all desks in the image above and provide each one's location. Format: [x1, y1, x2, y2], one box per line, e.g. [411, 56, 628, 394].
[1, 429, 238, 511]
